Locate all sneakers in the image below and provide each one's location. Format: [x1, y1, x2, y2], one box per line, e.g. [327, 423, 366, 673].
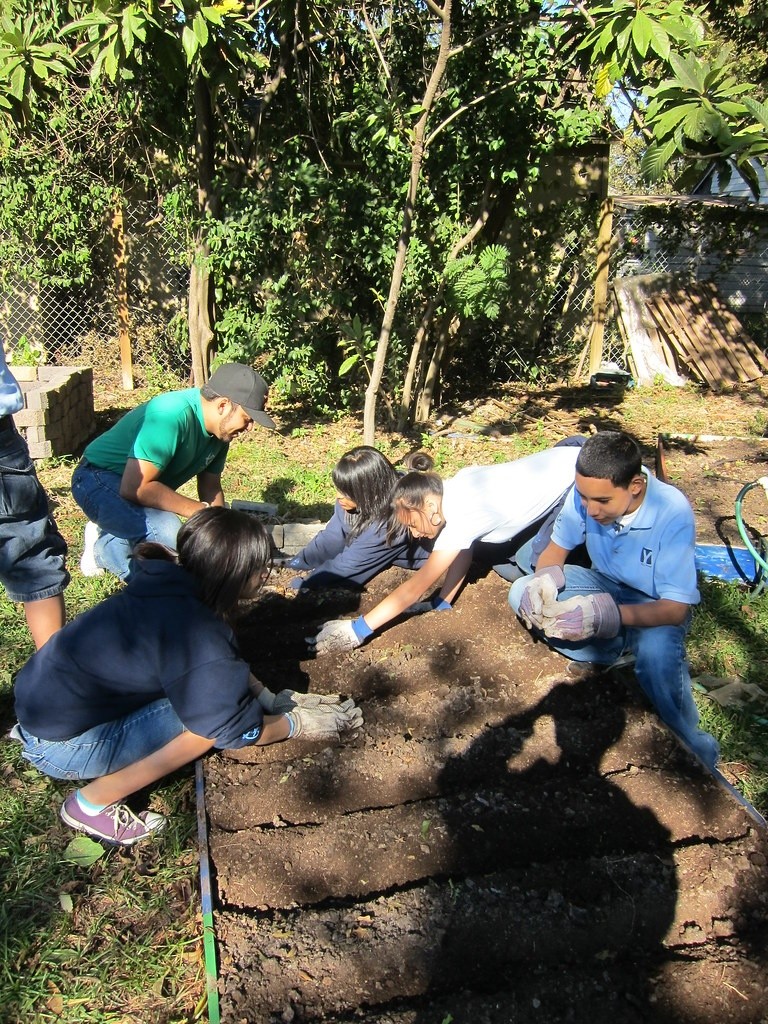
[566, 645, 637, 679]
[81, 521, 105, 577]
[61, 790, 167, 845]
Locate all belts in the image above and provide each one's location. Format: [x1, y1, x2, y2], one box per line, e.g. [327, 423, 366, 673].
[0, 414, 15, 432]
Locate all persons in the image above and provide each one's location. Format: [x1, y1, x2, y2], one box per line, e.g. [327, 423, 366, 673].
[0, 339, 72, 649]
[10, 504, 363, 846]
[507, 431, 719, 775]
[285, 436, 591, 659]
[69, 362, 277, 585]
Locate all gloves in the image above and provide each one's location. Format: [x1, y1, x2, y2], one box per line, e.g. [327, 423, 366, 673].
[273, 689, 364, 743]
[518, 565, 565, 630]
[304, 615, 374, 657]
[403, 596, 452, 613]
[541, 593, 620, 641]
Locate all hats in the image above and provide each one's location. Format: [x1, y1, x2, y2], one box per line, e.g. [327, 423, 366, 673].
[208, 363, 276, 428]
